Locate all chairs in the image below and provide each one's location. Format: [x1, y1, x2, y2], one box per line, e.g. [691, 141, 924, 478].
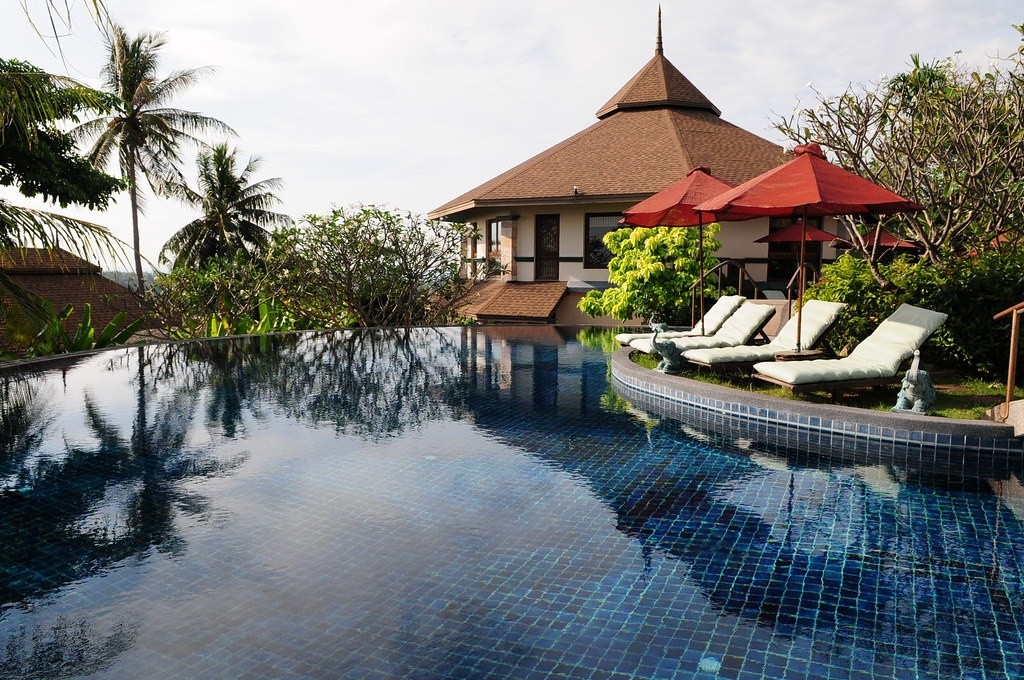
[748, 302, 949, 397]
[614, 295, 746, 346]
[681, 298, 851, 376]
[628, 301, 777, 355]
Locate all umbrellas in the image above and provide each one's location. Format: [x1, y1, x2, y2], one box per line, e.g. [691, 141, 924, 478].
[844, 224, 918, 256]
[970, 229, 1024, 258]
[692, 141, 921, 352]
[616, 165, 764, 336]
[749, 215, 844, 243]
